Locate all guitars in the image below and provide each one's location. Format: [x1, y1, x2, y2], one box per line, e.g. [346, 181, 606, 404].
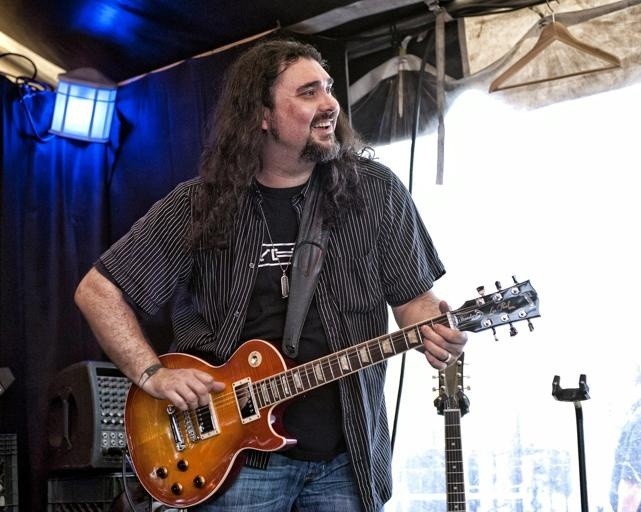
[432, 351, 470, 511]
[124, 275, 541, 508]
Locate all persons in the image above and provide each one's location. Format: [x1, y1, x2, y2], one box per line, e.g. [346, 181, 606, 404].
[74, 36, 468, 512]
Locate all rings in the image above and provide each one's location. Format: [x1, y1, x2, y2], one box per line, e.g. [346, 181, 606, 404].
[444, 352, 452, 363]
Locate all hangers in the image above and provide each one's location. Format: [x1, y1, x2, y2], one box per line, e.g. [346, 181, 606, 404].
[447, 0, 641, 96]
[488, 1, 623, 94]
[345, 30, 457, 108]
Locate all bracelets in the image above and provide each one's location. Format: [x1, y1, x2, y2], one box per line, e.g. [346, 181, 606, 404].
[136, 363, 165, 389]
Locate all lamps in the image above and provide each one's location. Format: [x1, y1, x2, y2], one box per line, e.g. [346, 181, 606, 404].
[52, 65, 118, 144]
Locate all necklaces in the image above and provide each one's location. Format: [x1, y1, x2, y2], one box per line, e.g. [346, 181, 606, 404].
[258, 193, 297, 299]
